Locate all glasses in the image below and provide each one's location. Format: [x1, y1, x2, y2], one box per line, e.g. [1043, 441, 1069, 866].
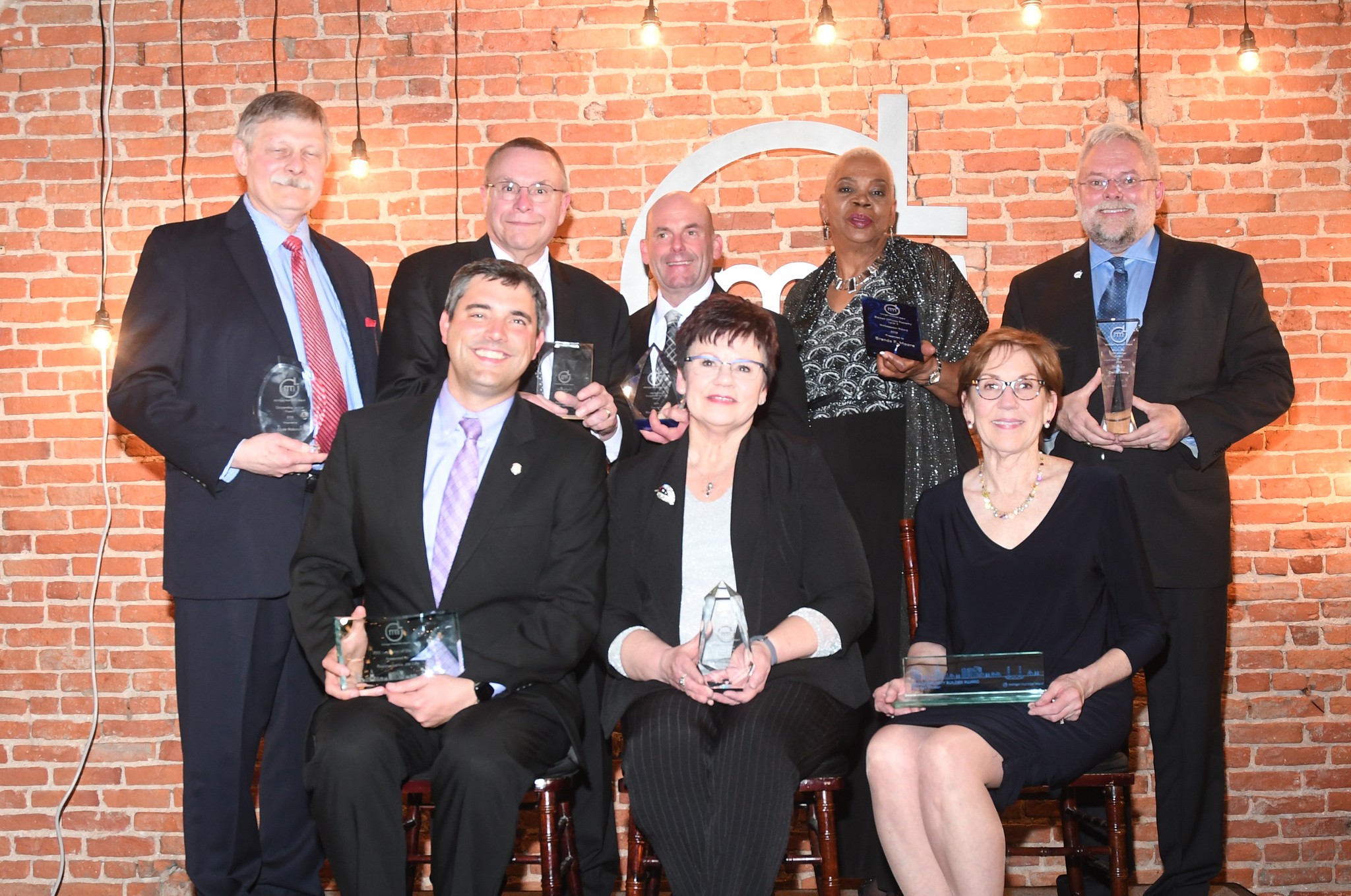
[483, 181, 565, 203]
[960, 378, 1051, 401]
[678, 355, 766, 383]
[1075, 175, 1158, 194]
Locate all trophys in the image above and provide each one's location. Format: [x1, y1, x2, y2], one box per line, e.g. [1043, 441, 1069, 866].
[860, 295, 925, 364]
[256, 364, 327, 444]
[333, 608, 466, 692]
[535, 340, 595, 420]
[1093, 318, 1145, 434]
[697, 579, 756, 693]
[892, 651, 1046, 710]
[619, 341, 686, 432]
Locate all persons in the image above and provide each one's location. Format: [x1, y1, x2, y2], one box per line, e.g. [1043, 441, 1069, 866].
[375, 137, 644, 896]
[999, 121, 1297, 896]
[288, 258, 610, 896]
[592, 294, 882, 896]
[865, 327, 1162, 896]
[629, 190, 814, 447]
[783, 146, 989, 896]
[107, 90, 380, 896]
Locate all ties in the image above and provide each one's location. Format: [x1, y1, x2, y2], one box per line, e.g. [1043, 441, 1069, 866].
[283, 233, 347, 459]
[1097, 256, 1131, 357]
[431, 417, 485, 606]
[654, 309, 682, 409]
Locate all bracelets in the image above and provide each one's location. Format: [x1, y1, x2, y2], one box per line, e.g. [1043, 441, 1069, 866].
[749, 634, 778, 666]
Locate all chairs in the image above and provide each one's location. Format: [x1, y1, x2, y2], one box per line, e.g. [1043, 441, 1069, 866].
[399, 519, 1138, 896]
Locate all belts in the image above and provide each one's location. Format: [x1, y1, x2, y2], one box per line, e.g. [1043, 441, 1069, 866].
[305, 475, 320, 492]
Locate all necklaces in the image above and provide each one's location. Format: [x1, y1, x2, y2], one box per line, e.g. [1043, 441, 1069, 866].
[689, 456, 735, 498]
[830, 251, 885, 294]
[977, 451, 1045, 519]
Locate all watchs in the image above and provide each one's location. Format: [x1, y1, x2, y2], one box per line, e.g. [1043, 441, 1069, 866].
[473, 681, 494, 703]
[919, 353, 943, 386]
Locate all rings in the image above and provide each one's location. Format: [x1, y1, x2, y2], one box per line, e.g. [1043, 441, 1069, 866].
[679, 674, 687, 686]
[603, 408, 611, 418]
[1147, 444, 1152, 449]
[1059, 718, 1064, 724]
[1084, 440, 1092, 448]
